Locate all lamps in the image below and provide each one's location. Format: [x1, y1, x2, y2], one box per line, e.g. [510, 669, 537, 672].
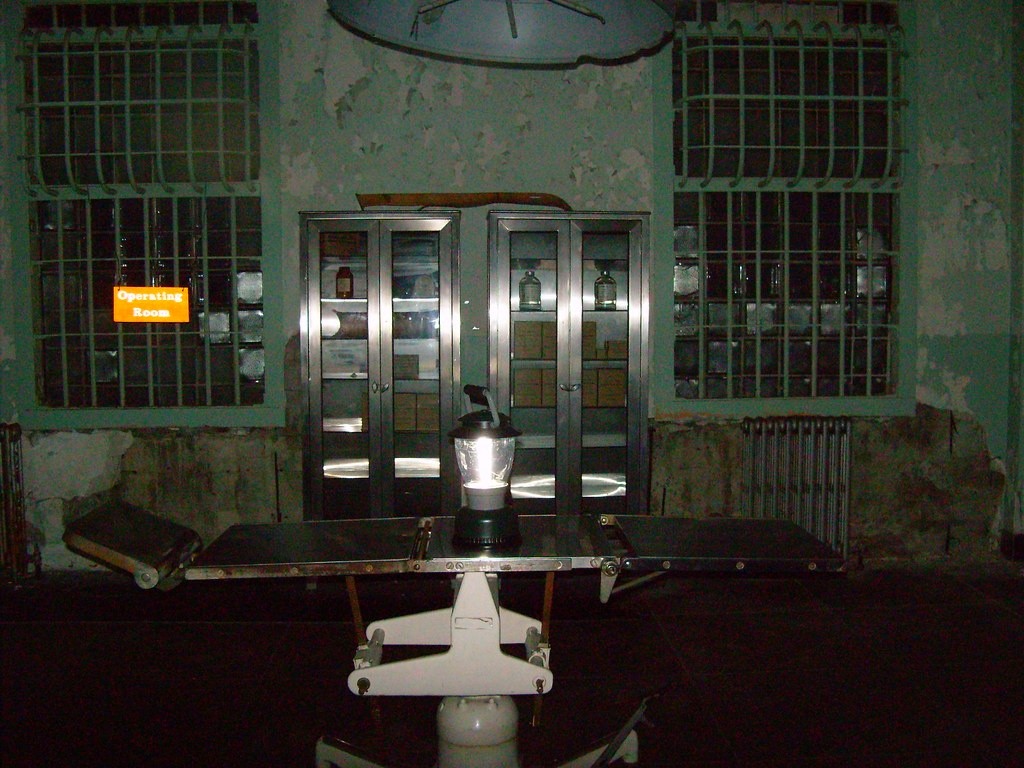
[447, 383, 522, 550]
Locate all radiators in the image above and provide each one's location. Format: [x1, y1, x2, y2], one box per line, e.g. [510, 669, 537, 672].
[739, 414, 851, 561]
[0, 423, 30, 591]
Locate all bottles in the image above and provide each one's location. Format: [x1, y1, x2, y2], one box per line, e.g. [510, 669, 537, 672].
[519, 271, 542, 311]
[335, 263, 355, 299]
[593, 270, 617, 312]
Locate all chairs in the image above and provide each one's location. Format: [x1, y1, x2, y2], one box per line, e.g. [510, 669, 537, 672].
[59, 500, 847, 738]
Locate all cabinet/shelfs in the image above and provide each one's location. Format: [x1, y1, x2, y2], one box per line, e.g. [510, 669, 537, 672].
[485, 209, 652, 517]
[296, 209, 463, 522]
